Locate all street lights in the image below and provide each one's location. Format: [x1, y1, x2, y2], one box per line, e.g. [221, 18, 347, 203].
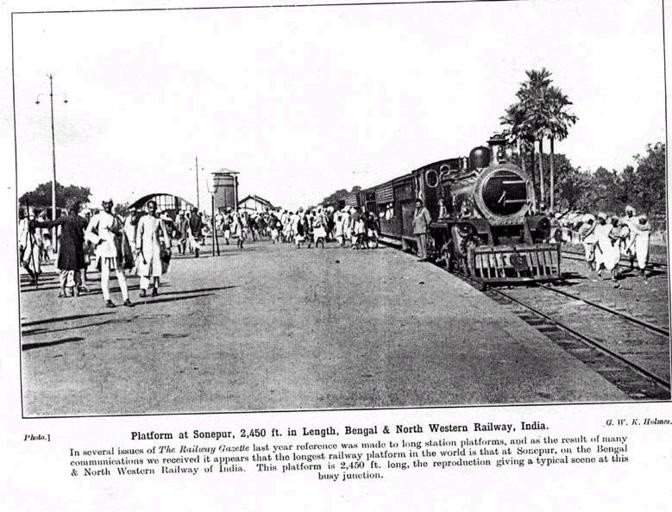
[190, 156, 205, 212]
[34, 68, 69, 254]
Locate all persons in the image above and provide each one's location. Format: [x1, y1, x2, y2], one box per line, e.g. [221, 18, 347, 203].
[411, 198, 432, 261]
[18, 193, 181, 308]
[541, 202, 653, 288]
[171, 202, 384, 259]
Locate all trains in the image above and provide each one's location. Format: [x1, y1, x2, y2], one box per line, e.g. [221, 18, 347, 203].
[318, 133, 561, 286]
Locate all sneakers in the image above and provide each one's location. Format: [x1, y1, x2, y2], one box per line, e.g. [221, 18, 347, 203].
[150, 286, 159, 297]
[123, 298, 135, 307]
[138, 288, 147, 298]
[105, 299, 116, 308]
[173, 234, 428, 263]
[56, 285, 80, 298]
